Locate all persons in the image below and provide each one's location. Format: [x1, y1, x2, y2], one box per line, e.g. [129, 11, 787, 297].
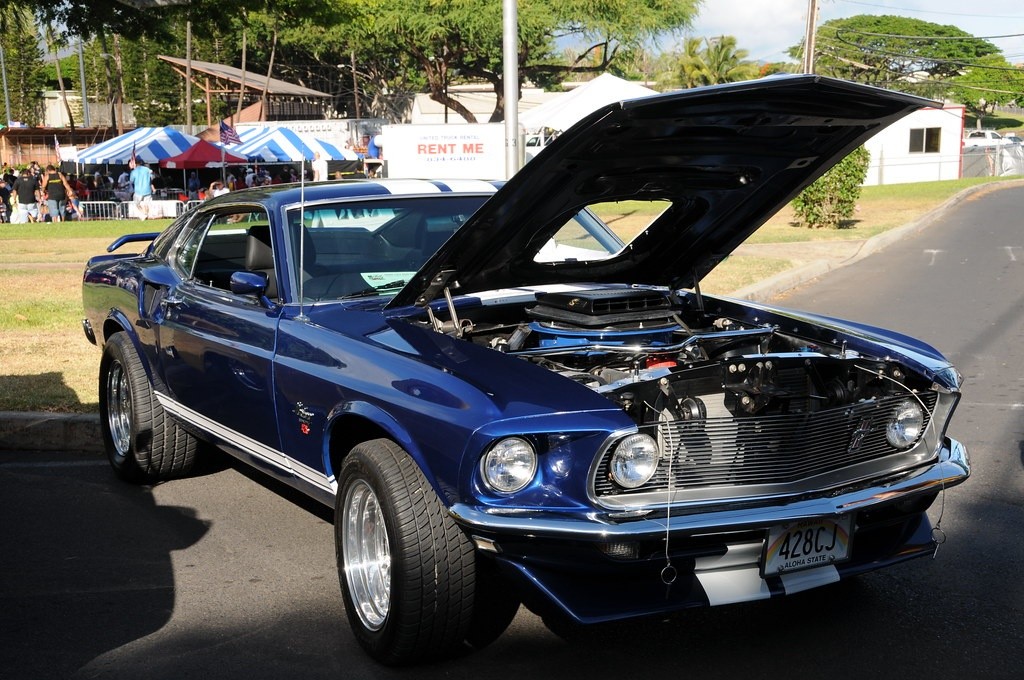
[0, 153, 376, 224]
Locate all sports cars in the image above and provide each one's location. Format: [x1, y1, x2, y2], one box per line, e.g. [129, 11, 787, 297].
[77, 73, 973, 656]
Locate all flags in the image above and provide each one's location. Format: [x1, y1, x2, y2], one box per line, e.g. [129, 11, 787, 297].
[219, 118, 244, 144]
[130, 142, 136, 169]
[55, 135, 61, 168]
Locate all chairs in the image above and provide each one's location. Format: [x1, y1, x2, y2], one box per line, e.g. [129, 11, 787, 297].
[412, 230, 455, 270]
[244, 222, 330, 299]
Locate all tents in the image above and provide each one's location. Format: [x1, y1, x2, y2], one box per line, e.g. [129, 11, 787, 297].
[500, 72, 661, 146]
[67, 127, 365, 204]
